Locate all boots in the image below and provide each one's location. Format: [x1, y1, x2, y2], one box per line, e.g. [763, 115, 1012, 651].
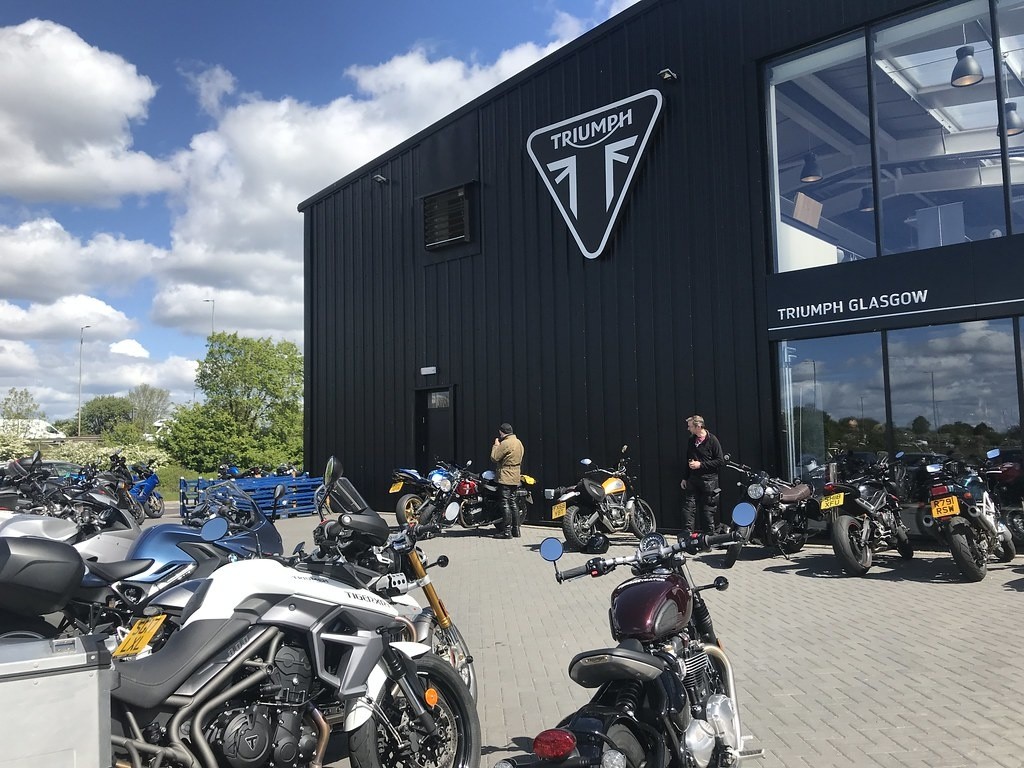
[511, 512, 521, 537]
[494, 511, 513, 539]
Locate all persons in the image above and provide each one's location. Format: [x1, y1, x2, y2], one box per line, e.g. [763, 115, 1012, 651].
[110, 454, 132, 482]
[680, 415, 724, 541]
[490, 423, 524, 539]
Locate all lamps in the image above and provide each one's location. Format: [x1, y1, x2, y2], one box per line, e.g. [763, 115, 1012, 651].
[372, 175, 389, 184]
[799, 74, 823, 183]
[657, 68, 677, 81]
[996, 60, 1024, 137]
[858, 123, 875, 213]
[951, 24, 984, 88]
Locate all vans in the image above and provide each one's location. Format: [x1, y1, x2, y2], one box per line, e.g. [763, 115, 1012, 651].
[142, 419, 176, 442]
[0, 417, 67, 441]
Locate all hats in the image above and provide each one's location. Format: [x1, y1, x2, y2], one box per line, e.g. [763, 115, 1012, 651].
[499, 423, 513, 434]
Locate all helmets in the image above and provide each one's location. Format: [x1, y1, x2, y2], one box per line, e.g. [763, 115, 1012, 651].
[586, 532, 609, 554]
[482, 470, 496, 480]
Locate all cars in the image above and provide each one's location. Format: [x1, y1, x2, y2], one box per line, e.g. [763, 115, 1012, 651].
[799, 446, 1024, 509]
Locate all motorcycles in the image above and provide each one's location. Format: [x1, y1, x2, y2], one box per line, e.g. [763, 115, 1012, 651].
[0, 440, 538, 768]
[819, 451, 914, 577]
[492, 499, 766, 767]
[552, 443, 656, 551]
[720, 453, 816, 570]
[924, 447, 1016, 583]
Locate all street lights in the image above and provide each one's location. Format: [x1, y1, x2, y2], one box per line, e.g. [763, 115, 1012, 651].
[78, 325, 92, 437]
[203, 298, 216, 347]
[860, 396, 869, 436]
[924, 371, 939, 437]
